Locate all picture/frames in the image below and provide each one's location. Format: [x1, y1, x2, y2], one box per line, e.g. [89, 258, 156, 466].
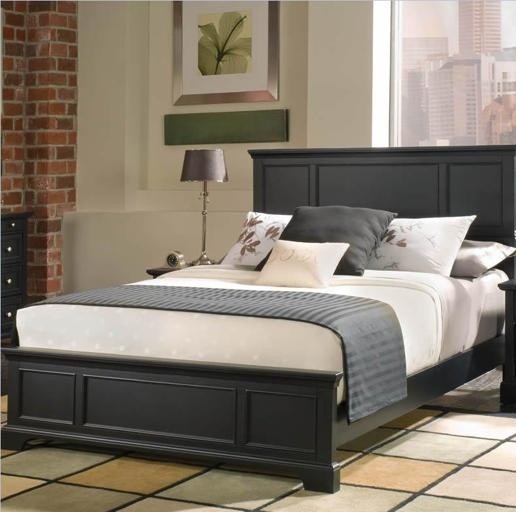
[172, 0, 280, 105]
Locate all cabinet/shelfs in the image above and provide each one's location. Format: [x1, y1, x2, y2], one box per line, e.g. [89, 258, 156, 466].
[1, 211, 35, 340]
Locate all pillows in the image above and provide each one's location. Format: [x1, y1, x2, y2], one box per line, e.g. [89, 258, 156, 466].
[220, 205, 516, 290]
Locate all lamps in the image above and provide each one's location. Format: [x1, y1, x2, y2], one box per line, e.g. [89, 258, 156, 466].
[179, 148, 229, 266]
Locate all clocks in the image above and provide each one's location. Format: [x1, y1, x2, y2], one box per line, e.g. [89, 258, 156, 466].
[167, 252, 186, 266]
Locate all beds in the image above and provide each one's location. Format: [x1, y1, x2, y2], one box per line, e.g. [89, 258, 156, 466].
[1, 143, 516, 494]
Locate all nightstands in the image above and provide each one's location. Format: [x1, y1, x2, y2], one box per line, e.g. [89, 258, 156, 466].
[146, 265, 181, 279]
[497, 279, 516, 413]
